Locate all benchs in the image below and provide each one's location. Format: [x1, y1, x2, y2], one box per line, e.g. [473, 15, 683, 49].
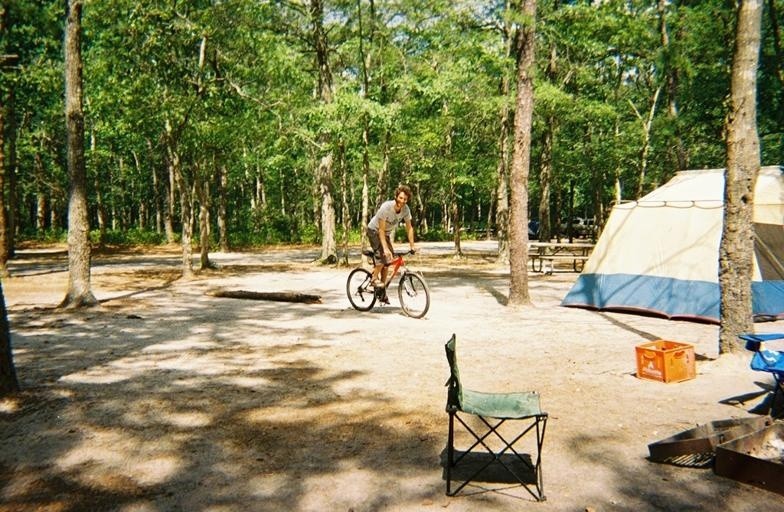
[528, 255, 577, 271]
[539, 256, 589, 276]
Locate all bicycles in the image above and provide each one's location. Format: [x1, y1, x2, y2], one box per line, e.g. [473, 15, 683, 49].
[346, 250, 430, 318]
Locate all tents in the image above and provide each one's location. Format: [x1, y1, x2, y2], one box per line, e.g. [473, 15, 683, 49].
[559, 163, 784, 326]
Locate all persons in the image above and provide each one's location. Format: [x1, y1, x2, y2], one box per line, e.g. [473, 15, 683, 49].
[364, 185, 418, 305]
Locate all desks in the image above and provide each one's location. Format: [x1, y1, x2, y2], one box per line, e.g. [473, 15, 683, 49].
[531, 243, 595, 273]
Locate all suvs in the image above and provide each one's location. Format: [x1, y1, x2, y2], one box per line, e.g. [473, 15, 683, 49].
[561, 217, 597, 236]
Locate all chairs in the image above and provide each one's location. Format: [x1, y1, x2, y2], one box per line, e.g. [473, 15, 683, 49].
[444, 334, 546, 501]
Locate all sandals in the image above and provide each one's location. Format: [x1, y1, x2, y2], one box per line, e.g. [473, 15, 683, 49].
[378, 290, 390, 304]
[369, 278, 384, 287]
